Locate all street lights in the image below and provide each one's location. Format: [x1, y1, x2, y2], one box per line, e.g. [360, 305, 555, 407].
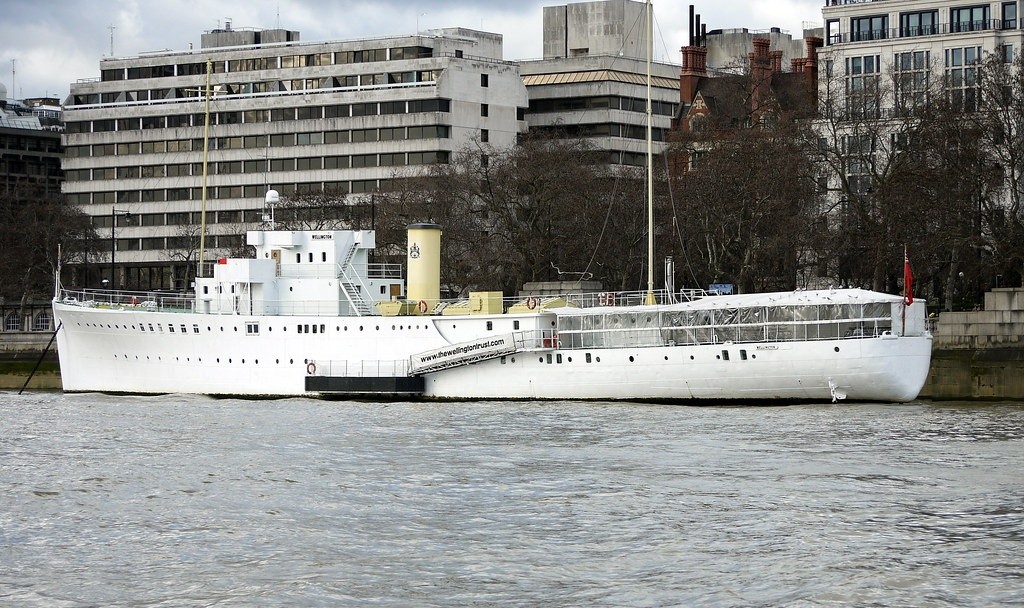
[112, 206, 131, 292]
[371, 193, 389, 278]
[959, 272, 964, 312]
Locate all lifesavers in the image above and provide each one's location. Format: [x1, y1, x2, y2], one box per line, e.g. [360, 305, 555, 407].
[553, 335, 557, 348]
[527, 297, 536, 309]
[598, 293, 607, 306]
[419, 300, 428, 314]
[607, 294, 614, 306]
[307, 363, 316, 374]
[544, 339, 552, 348]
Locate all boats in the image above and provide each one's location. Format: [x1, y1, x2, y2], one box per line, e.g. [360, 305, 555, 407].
[52, 0, 933, 401]
